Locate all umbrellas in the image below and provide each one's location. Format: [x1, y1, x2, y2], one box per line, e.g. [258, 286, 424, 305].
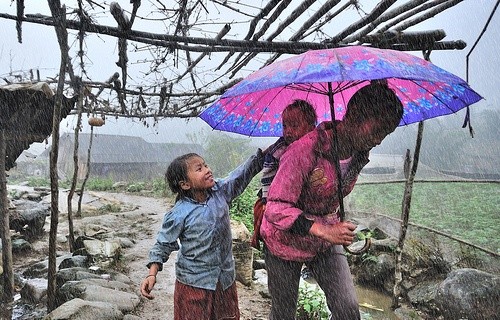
[197, 46, 486, 255]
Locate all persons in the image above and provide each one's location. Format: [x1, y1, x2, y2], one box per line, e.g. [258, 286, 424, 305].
[251, 99, 317, 250]
[141, 144, 274, 320]
[260, 84, 403, 320]
